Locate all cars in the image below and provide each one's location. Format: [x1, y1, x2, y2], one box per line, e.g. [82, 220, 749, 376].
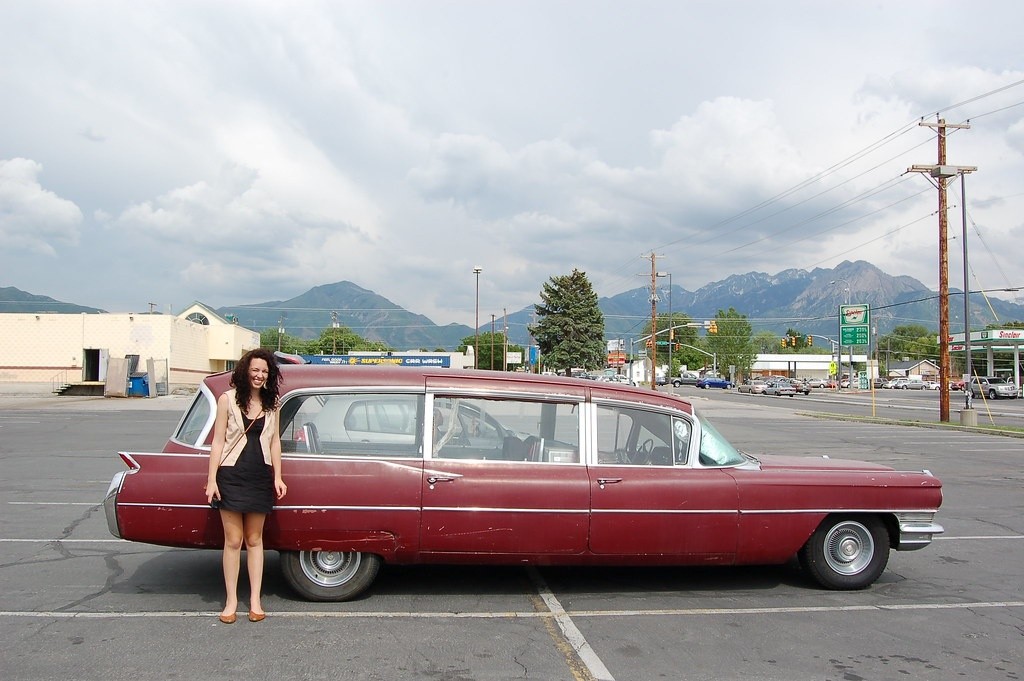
[784, 378, 812, 395]
[737, 380, 770, 394]
[655, 372, 964, 389]
[516, 366, 630, 385]
[103, 351, 945, 600]
[762, 382, 797, 397]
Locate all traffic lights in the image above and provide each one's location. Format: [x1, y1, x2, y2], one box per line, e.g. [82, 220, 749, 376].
[792, 337, 796, 347]
[781, 338, 787, 348]
[807, 336, 812, 346]
[675, 343, 679, 351]
[646, 339, 653, 349]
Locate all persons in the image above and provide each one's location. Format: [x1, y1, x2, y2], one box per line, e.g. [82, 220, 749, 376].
[433, 408, 463, 446]
[204, 349, 289, 624]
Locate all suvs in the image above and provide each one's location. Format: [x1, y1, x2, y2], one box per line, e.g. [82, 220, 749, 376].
[970, 375, 1018, 400]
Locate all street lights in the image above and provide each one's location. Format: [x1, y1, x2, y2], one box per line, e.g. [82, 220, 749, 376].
[637, 272, 673, 383]
[330, 311, 339, 354]
[830, 277, 853, 388]
[278, 315, 288, 351]
[473, 267, 482, 369]
[148, 301, 157, 313]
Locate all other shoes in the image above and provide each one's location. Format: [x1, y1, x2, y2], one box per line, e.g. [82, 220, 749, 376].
[220, 612, 236, 623]
[249, 611, 264, 622]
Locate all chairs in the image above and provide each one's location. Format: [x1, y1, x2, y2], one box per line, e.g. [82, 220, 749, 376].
[502, 436, 522, 461]
[521, 435, 545, 462]
[296, 420, 325, 455]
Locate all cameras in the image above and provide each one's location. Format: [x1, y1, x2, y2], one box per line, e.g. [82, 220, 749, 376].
[210, 495, 221, 509]
